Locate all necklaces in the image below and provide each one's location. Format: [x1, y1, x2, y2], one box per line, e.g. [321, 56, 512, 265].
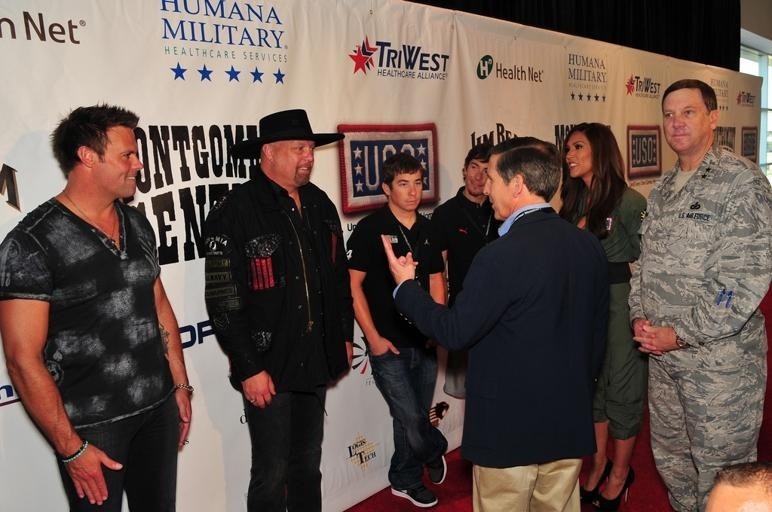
[64, 189, 116, 245]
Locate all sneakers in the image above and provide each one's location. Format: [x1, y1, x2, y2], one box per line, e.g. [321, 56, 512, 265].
[426, 456, 447, 485]
[391, 480, 437, 507]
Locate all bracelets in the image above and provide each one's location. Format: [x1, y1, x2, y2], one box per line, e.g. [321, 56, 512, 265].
[173, 383, 194, 391]
[60, 440, 89, 462]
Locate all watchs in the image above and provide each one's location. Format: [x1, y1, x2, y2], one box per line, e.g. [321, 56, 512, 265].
[675, 336, 687, 350]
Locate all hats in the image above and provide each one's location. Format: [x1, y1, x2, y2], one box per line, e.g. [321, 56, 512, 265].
[230, 109, 344, 158]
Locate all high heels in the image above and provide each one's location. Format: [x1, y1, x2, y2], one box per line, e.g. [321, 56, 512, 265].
[591, 466, 634, 512]
[580, 458, 613, 505]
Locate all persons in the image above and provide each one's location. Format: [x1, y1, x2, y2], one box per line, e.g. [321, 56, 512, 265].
[627, 81, 772, 511]
[347, 153, 448, 507]
[704, 463, 772, 511]
[0, 100, 194, 512]
[433, 145, 504, 296]
[203, 108, 354, 512]
[561, 123, 648, 512]
[381, 137, 612, 512]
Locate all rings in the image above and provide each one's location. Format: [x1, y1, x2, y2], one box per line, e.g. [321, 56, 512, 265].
[250, 400, 256, 403]
